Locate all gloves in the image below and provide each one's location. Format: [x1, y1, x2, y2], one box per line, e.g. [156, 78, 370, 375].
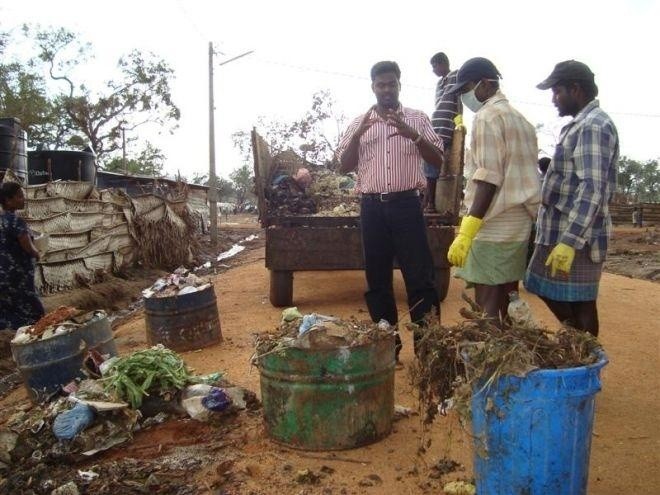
[447, 215, 484, 268]
[545, 242, 576, 281]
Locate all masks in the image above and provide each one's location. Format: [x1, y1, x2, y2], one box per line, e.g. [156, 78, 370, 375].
[460, 77, 487, 112]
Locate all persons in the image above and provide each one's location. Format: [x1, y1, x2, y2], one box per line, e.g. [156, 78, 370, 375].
[333, 60, 445, 375]
[446, 55, 543, 340]
[0, 179, 44, 330]
[632, 206, 640, 228]
[522, 59, 621, 340]
[418, 50, 460, 212]
[527, 155, 553, 271]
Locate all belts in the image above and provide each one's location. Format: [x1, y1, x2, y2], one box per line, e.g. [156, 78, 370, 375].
[362, 189, 420, 202]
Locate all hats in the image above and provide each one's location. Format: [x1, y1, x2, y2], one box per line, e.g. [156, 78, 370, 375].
[447, 57, 502, 94]
[536, 60, 594, 90]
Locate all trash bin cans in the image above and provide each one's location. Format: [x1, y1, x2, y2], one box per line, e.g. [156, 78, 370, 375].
[10, 313, 122, 409]
[257, 331, 397, 452]
[460, 348, 609, 495]
[143, 282, 222, 353]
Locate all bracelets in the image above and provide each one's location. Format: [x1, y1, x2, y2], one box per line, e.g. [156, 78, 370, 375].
[410, 135, 422, 144]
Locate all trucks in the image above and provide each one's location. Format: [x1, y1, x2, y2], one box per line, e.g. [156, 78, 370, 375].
[250, 126, 467, 307]
